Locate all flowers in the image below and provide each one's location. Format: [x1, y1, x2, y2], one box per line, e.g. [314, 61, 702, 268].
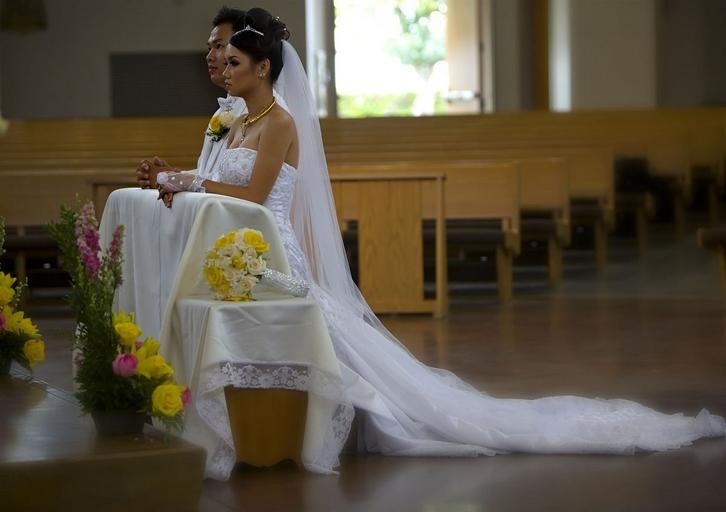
[0, 215, 45, 386]
[44, 192, 195, 447]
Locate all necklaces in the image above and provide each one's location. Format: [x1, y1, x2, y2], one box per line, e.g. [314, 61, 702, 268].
[236, 95, 277, 144]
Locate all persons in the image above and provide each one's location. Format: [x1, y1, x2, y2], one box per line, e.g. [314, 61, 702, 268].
[154, 6, 316, 457]
[134, 6, 258, 193]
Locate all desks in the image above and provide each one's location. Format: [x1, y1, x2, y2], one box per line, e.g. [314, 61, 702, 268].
[177, 294, 324, 474]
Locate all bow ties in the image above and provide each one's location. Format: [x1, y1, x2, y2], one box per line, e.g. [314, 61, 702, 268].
[216, 96, 236, 110]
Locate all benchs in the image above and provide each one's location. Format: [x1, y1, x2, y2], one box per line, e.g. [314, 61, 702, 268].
[1, 105, 725, 319]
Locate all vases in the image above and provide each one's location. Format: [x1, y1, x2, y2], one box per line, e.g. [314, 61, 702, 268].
[90, 403, 146, 436]
